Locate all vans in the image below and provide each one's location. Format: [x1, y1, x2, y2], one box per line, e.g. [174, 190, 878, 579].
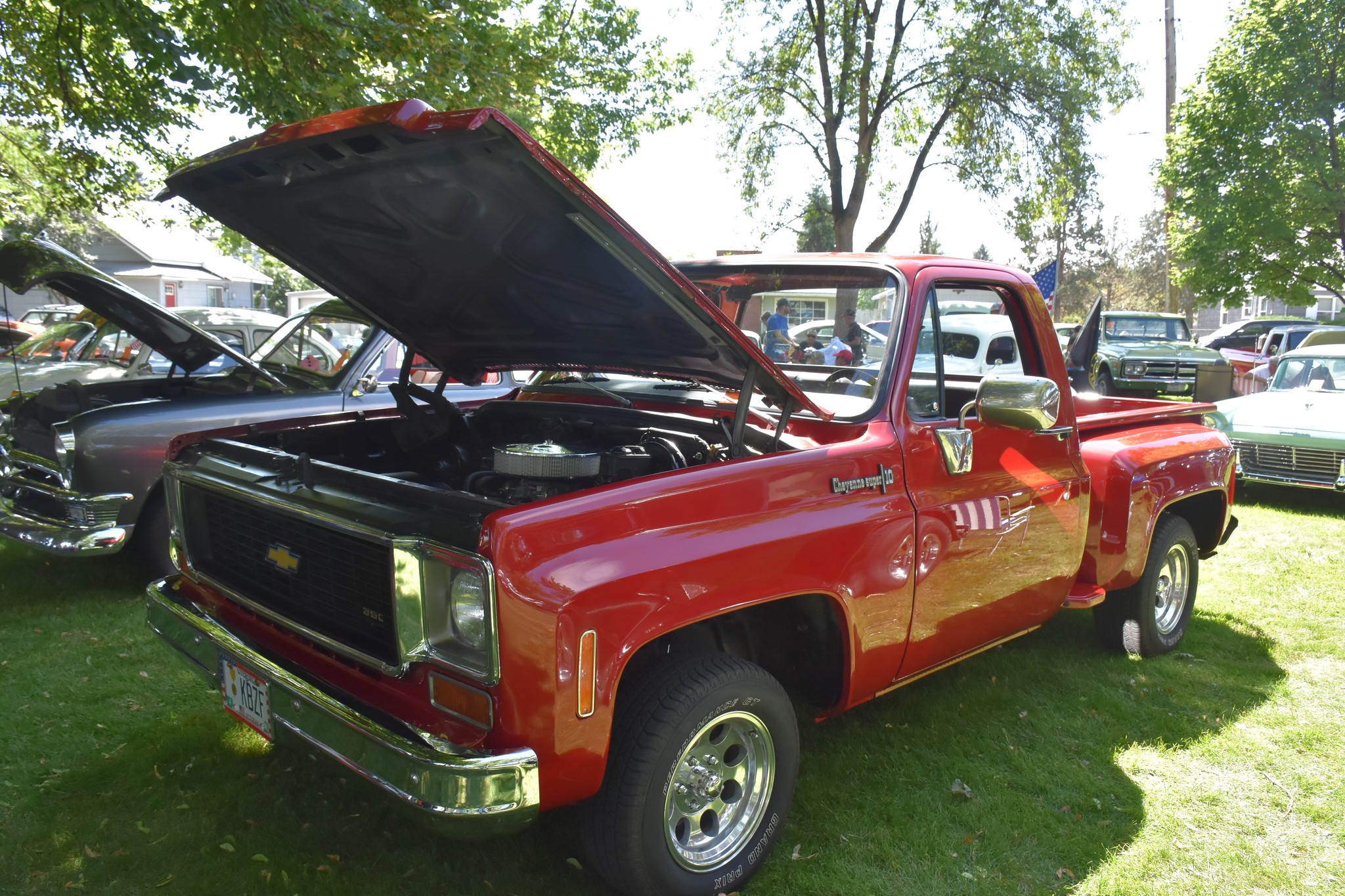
[1200, 318, 1318, 349]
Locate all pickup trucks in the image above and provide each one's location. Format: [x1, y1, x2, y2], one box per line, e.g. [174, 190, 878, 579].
[1218, 325, 1345, 397]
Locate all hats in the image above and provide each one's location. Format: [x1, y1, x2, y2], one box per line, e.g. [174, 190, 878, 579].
[833, 349, 853, 361]
[777, 298, 794, 306]
[804, 351, 825, 364]
[840, 310, 855, 317]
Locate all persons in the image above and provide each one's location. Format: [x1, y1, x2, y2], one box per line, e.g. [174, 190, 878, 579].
[790, 330, 825, 365]
[839, 309, 862, 367]
[322, 328, 343, 359]
[1067, 325, 1084, 368]
[986, 303, 1001, 351]
[803, 350, 825, 366]
[760, 311, 772, 353]
[1244, 356, 1279, 392]
[833, 349, 854, 394]
[765, 298, 800, 363]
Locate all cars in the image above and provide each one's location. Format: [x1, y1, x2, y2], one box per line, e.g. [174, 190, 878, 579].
[138, 99, 1243, 895]
[0, 238, 537, 582]
[782, 300, 1200, 399]
[1201, 344, 1345, 492]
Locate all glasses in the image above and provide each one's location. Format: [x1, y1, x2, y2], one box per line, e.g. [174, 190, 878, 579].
[807, 334, 816, 338]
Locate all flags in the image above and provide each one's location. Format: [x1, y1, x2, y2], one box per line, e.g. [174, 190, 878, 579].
[999, 261, 1057, 317]
[1071, 297, 1102, 391]
